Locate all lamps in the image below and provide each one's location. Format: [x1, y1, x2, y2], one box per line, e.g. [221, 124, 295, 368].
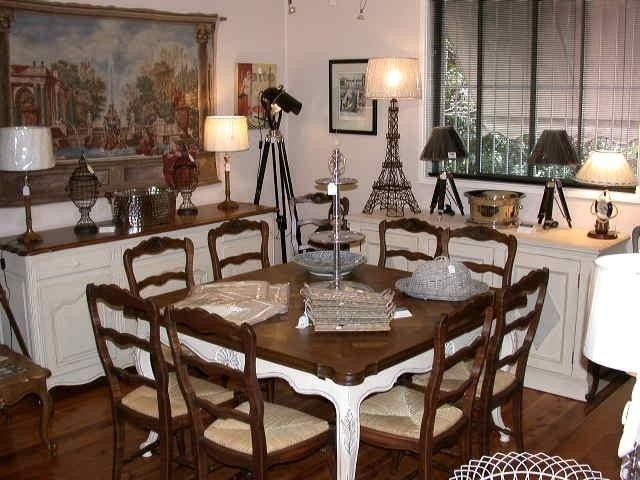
[419, 126, 468, 217]
[581, 252, 640, 377]
[0, 125, 57, 243]
[527, 129, 579, 229]
[574, 150, 637, 239]
[202, 114, 250, 211]
[362, 57, 421, 217]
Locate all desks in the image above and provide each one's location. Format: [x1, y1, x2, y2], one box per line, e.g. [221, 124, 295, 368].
[2, 200, 280, 406]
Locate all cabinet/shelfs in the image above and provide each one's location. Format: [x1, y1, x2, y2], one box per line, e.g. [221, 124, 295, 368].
[359, 221, 580, 377]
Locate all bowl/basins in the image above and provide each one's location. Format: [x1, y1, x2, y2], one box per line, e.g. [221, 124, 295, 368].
[103, 185, 180, 228]
[462, 188, 528, 227]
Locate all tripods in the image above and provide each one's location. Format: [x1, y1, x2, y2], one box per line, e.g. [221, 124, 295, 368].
[254, 137, 302, 263]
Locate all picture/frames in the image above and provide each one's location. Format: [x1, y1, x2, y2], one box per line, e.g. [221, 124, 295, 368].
[327, 58, 378, 136]
[0, 0, 225, 208]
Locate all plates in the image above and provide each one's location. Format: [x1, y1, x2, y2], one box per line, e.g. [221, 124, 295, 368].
[292, 249, 366, 278]
[394, 275, 491, 303]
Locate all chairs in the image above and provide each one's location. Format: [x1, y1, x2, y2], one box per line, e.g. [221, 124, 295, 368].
[83, 282, 235, 480]
[164, 304, 337, 480]
[359, 286, 497, 480]
[0, 344, 56, 459]
[394, 267, 549, 480]
[207, 218, 270, 281]
[123, 235, 195, 302]
[441, 227, 517, 288]
[378, 219, 443, 269]
[584, 224, 640, 400]
[288, 191, 353, 252]
